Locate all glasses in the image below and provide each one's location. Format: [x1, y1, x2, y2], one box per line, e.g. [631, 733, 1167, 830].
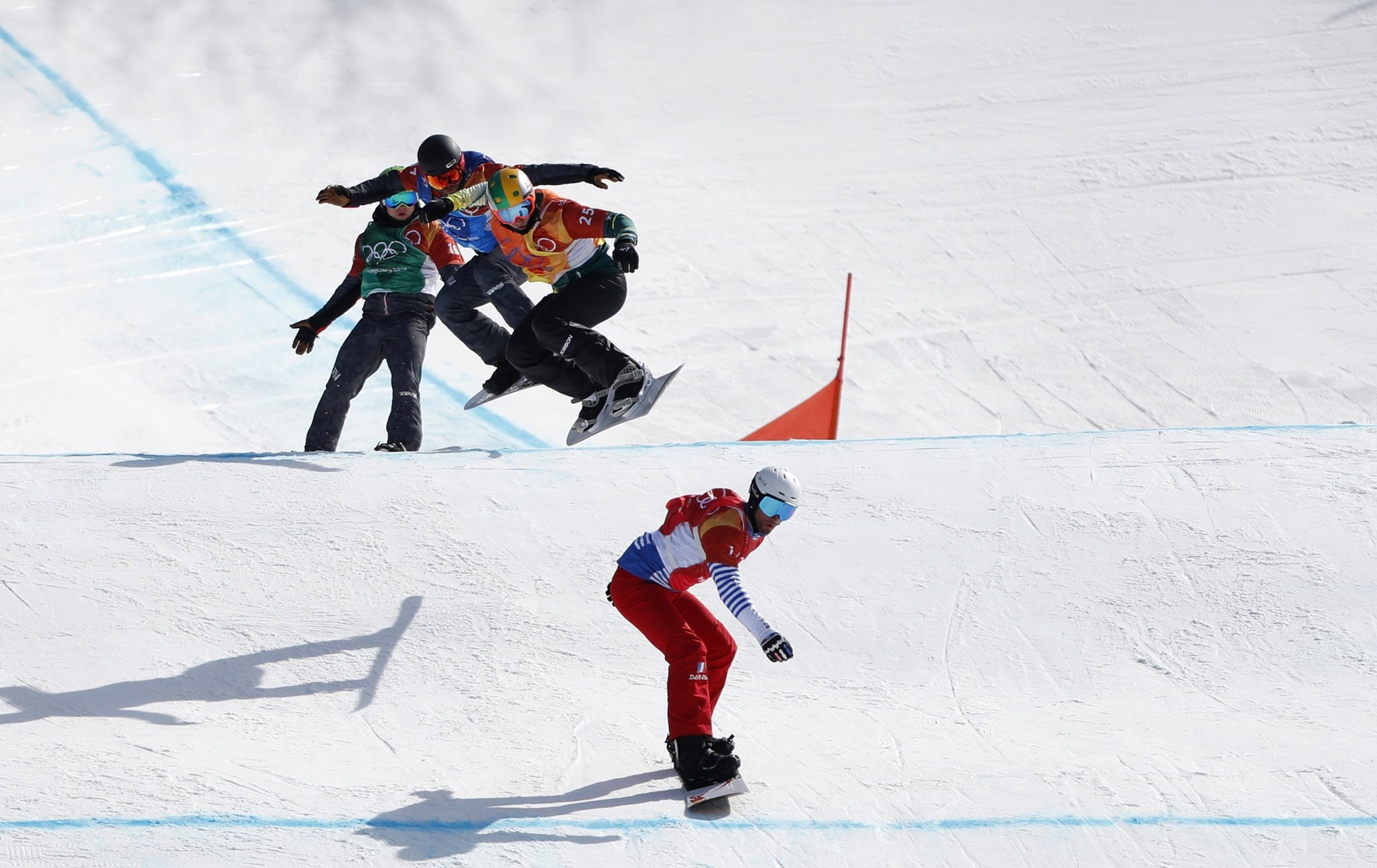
[422, 167, 462, 189]
[383, 193, 418, 208]
[493, 196, 534, 226]
[756, 495, 797, 521]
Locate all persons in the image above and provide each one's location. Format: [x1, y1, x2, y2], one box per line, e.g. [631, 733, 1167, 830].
[316, 135, 626, 395]
[606, 468, 800, 782]
[289, 167, 466, 452]
[419, 166, 650, 435]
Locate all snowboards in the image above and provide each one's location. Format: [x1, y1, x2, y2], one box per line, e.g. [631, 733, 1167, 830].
[462, 375, 544, 411]
[566, 361, 687, 447]
[679, 750, 751, 809]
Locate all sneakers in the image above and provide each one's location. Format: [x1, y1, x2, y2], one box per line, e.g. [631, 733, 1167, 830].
[664, 734, 734, 764]
[483, 361, 516, 395]
[570, 390, 608, 433]
[609, 359, 643, 416]
[375, 441, 406, 452]
[674, 736, 741, 790]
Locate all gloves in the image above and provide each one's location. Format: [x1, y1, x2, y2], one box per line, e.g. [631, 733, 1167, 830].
[759, 631, 793, 663]
[288, 319, 316, 355]
[610, 243, 641, 273]
[584, 166, 625, 189]
[316, 182, 351, 207]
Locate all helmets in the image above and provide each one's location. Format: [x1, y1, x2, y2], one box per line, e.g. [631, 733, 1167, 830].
[416, 134, 462, 176]
[377, 165, 404, 177]
[751, 468, 799, 521]
[486, 166, 535, 231]
